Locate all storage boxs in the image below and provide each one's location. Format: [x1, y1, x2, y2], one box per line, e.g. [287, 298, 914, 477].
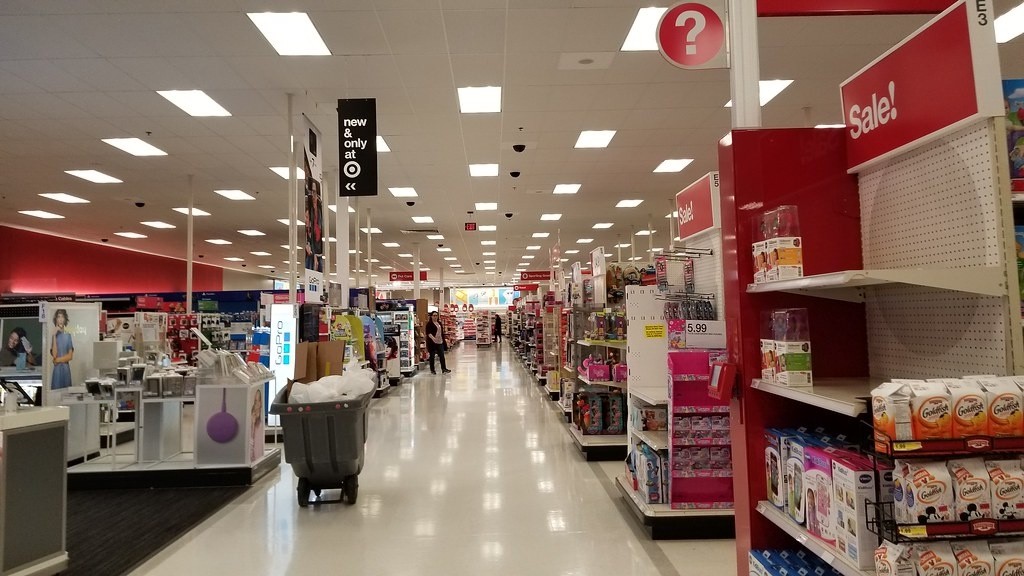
[589, 365, 627, 383]
[748, 548, 841, 576]
[548, 370, 561, 391]
[563, 381, 576, 408]
[764, 426, 896, 571]
[572, 392, 627, 436]
[632, 406, 668, 431]
[761, 306, 814, 389]
[591, 312, 625, 339]
[625, 441, 669, 504]
[751, 204, 804, 280]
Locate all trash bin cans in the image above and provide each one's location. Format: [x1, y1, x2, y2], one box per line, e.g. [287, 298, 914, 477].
[269, 376, 378, 507]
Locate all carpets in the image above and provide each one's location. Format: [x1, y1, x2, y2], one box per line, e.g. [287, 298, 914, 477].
[52, 483, 253, 576]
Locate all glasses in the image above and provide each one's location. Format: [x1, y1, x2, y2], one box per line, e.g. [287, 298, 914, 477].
[432, 315, 438, 316]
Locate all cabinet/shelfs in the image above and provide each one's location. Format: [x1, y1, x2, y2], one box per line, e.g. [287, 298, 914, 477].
[543, 305, 562, 400]
[0, 295, 391, 576]
[717, 0, 1024, 576]
[615, 170, 735, 541]
[568, 265, 658, 462]
[382, 295, 563, 385]
[557, 304, 573, 423]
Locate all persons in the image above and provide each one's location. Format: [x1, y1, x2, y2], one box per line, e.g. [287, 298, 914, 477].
[305, 180, 323, 272]
[759, 248, 780, 274]
[807, 489, 821, 536]
[250, 388, 264, 461]
[493, 314, 502, 343]
[768, 350, 787, 368]
[50, 309, 75, 390]
[0, 327, 38, 367]
[766, 462, 778, 504]
[788, 473, 798, 520]
[425, 311, 452, 375]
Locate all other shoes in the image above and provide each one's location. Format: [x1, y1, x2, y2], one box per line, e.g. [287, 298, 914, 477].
[431, 371, 436, 375]
[442, 368, 451, 374]
